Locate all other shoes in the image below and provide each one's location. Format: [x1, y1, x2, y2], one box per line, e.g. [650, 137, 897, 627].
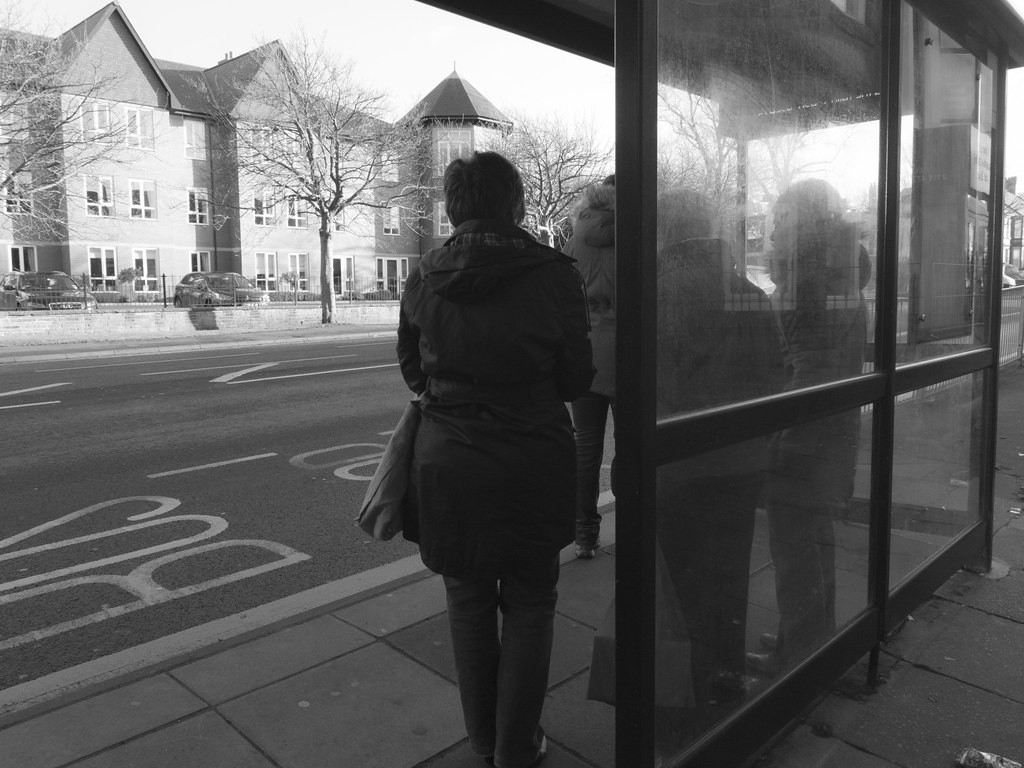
[745, 650, 781, 676]
[706, 682, 750, 706]
[484, 723, 547, 768]
[759, 634, 783, 651]
[575, 537, 600, 559]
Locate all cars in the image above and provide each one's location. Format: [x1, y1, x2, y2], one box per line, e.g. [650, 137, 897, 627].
[0, 270, 98, 313]
[173, 270, 271, 309]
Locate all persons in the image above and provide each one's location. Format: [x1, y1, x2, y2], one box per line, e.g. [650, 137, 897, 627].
[745, 179, 868, 677]
[393, 151, 597, 768]
[561, 174, 616, 559]
[657, 190, 792, 712]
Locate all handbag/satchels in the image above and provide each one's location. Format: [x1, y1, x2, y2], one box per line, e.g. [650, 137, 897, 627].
[356, 394, 423, 544]
